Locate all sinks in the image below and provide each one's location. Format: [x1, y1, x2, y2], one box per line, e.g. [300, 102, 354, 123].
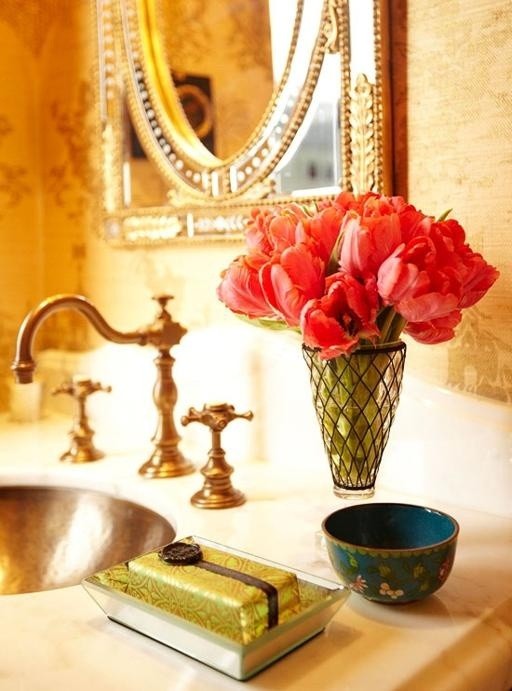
[0, 486, 177, 596]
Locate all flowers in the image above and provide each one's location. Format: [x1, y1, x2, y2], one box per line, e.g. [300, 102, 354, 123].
[213, 186, 501, 485]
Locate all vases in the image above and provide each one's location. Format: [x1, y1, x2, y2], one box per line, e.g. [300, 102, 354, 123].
[299, 338, 408, 501]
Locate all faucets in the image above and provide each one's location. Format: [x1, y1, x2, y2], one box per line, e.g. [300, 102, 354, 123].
[10, 294, 195, 477]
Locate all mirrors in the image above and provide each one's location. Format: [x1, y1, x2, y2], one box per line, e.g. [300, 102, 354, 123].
[93, 0, 396, 252]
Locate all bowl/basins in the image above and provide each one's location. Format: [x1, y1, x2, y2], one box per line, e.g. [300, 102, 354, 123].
[319, 502, 460, 606]
